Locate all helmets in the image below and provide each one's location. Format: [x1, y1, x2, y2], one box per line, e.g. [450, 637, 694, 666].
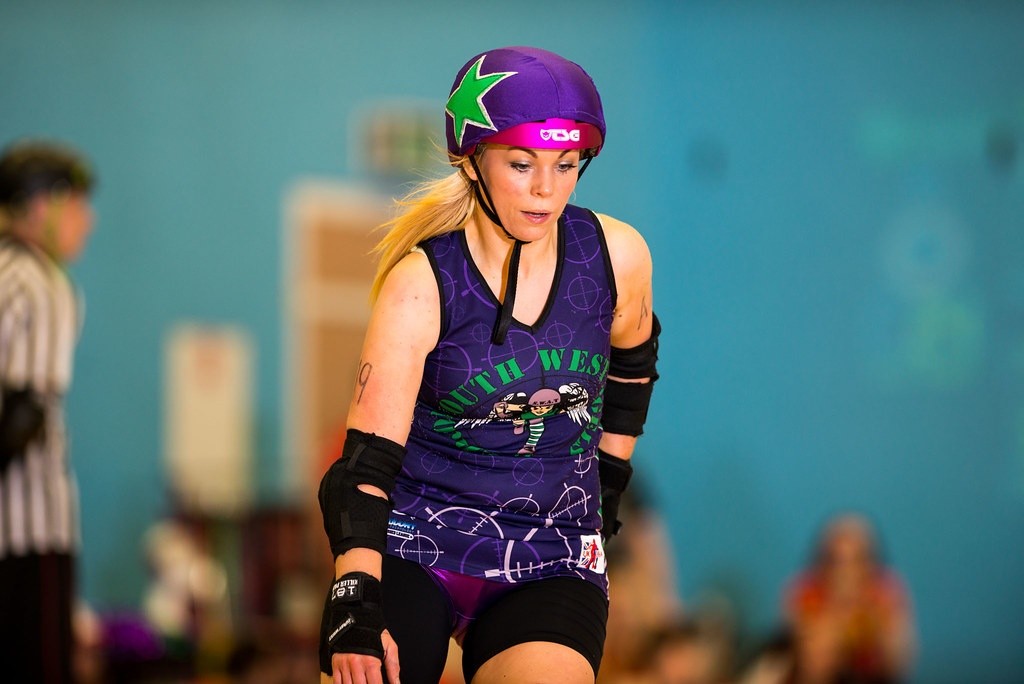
[1, 139, 93, 205]
[465, 116, 603, 161]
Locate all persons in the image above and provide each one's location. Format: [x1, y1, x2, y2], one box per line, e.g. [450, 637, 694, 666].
[612, 469, 920, 684]
[0, 135, 102, 684]
[310, 44, 661, 684]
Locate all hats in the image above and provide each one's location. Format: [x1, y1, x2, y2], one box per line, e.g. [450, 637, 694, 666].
[444, 47, 607, 165]
